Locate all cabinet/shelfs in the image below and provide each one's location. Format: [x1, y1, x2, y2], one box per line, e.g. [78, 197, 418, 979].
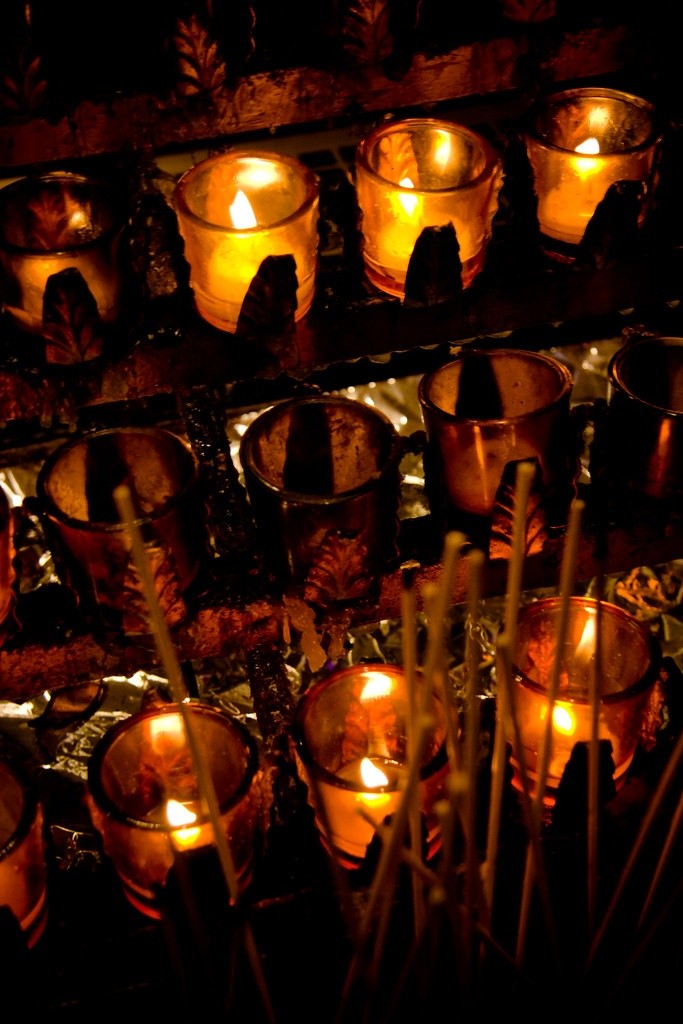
[0, 25, 683, 1024]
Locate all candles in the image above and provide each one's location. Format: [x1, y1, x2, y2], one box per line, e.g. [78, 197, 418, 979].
[0, 88, 683, 949]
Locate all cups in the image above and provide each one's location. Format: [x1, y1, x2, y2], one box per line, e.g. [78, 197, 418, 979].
[0, 84, 683, 945]
